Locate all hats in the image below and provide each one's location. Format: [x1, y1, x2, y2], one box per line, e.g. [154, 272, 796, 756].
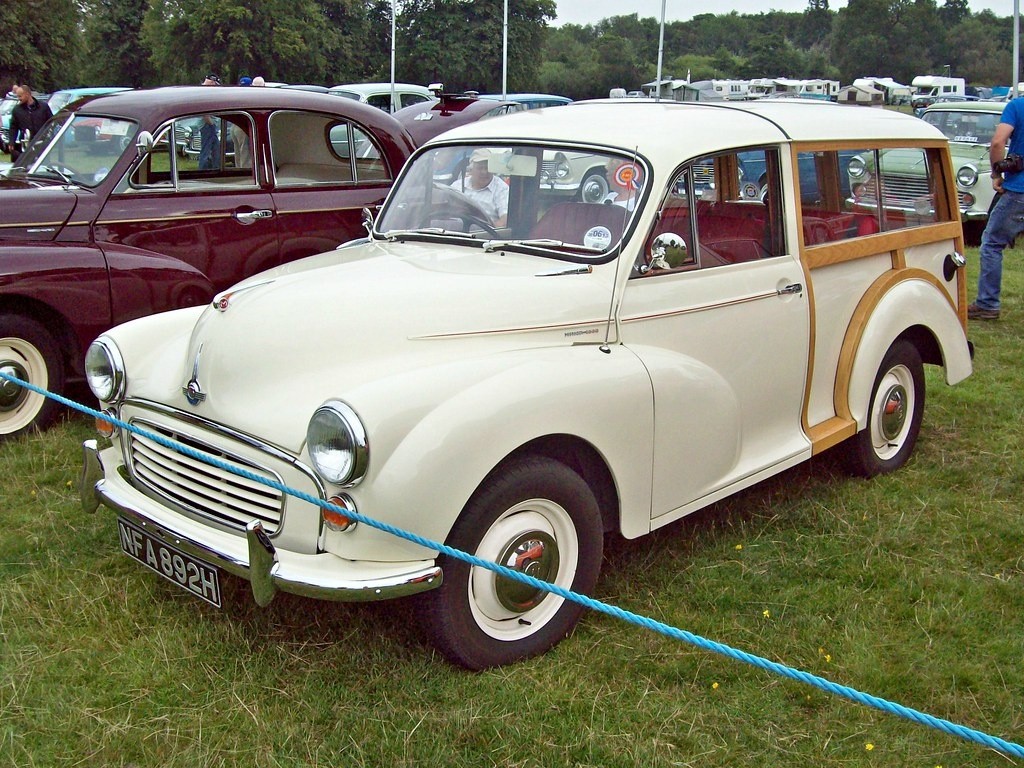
[240, 77, 252, 85]
[468, 148, 492, 162]
[253, 77, 265, 86]
[206, 74, 220, 85]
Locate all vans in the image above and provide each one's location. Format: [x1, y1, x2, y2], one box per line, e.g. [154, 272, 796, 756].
[47, 88, 138, 148]
[0, 85, 53, 153]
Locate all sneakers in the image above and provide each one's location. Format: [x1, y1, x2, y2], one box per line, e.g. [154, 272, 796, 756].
[968, 303, 1000, 318]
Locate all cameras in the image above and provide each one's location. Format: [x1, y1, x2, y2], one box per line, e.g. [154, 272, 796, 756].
[993, 152, 1024, 175]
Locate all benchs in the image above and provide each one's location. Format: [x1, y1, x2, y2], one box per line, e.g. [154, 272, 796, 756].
[277, 161, 385, 181]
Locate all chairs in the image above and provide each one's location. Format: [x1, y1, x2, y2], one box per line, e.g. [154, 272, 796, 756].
[669, 214, 767, 263]
[527, 203, 631, 241]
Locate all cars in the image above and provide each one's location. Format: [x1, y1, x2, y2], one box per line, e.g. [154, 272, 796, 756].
[914, 94, 980, 134]
[844, 100, 1017, 248]
[75, 98, 976, 670]
[486, 141, 642, 205]
[676, 146, 870, 208]
[0, 84, 491, 440]
[73, 116, 136, 153]
[324, 85, 435, 123]
[760, 91, 800, 99]
[477, 93, 572, 113]
[625, 91, 646, 98]
[183, 82, 331, 163]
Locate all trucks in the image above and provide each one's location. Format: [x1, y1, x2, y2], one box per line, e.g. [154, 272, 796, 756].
[910, 74, 966, 106]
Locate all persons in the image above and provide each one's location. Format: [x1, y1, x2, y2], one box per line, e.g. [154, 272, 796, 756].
[229, 77, 253, 168]
[967, 96, 1024, 320]
[190, 74, 220, 170]
[252, 76, 265, 87]
[8, 85, 55, 165]
[449, 149, 510, 227]
